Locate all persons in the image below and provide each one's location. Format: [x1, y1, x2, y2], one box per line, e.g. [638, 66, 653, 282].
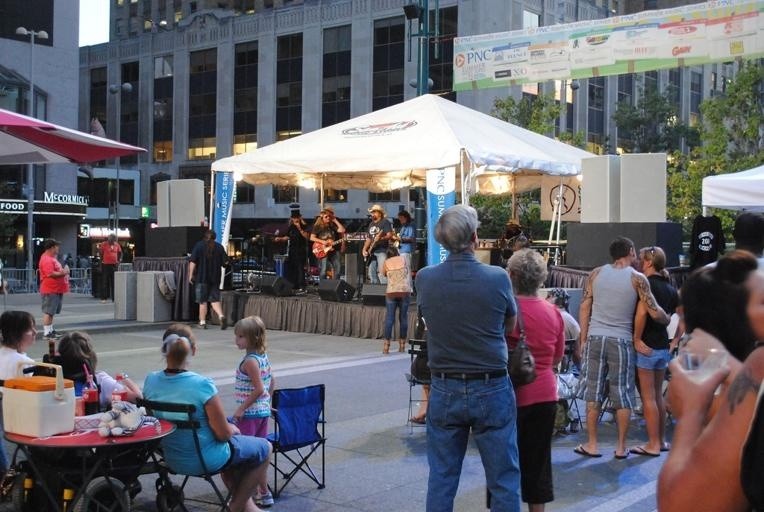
[682, 250, 764, 383]
[396, 210, 417, 277]
[233, 318, 274, 506]
[275, 209, 309, 291]
[362, 205, 392, 284]
[311, 207, 345, 281]
[573, 235, 672, 458]
[0, 310, 38, 472]
[190, 231, 232, 331]
[39, 239, 70, 341]
[144, 322, 273, 512]
[658, 348, 764, 512]
[381, 247, 413, 353]
[487, 248, 565, 512]
[98, 234, 123, 303]
[544, 288, 582, 373]
[497, 220, 527, 268]
[629, 248, 685, 457]
[418, 205, 522, 512]
[409, 316, 428, 424]
[59, 331, 143, 407]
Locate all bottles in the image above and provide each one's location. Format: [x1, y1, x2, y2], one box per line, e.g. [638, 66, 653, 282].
[112, 375, 128, 408]
[82, 374, 98, 416]
[572, 363, 579, 377]
[64, 263, 70, 284]
[570, 408, 579, 433]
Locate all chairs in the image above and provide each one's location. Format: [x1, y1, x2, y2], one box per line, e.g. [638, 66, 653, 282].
[564, 337, 583, 432]
[267, 384, 327, 498]
[406, 339, 430, 434]
[135, 398, 250, 511]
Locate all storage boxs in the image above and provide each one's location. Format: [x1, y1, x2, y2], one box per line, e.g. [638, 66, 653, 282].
[1, 362, 76, 437]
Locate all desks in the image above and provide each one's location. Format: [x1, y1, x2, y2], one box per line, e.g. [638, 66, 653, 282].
[6, 419, 176, 512]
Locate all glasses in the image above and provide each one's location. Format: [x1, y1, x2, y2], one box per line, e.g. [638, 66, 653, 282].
[321, 215, 332, 219]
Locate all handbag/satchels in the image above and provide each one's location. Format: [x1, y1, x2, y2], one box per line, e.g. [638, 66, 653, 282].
[503, 293, 538, 386]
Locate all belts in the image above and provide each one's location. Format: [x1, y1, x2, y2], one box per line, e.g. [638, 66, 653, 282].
[430, 367, 512, 382]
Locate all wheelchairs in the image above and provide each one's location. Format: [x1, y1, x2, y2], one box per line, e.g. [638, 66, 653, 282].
[11, 352, 184, 512]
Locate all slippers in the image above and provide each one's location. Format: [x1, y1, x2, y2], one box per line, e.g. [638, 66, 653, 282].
[574, 444, 602, 457]
[630, 444, 661, 457]
[614, 447, 629, 459]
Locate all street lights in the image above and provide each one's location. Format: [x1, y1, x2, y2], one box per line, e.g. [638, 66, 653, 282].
[108, 82, 133, 244]
[15, 26, 49, 291]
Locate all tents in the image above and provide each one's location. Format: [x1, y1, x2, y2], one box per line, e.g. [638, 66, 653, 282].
[207, 93, 600, 342]
[702, 164, 764, 217]
[0, 108, 148, 164]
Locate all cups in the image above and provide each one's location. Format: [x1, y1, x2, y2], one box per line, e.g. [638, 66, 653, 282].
[680, 350, 728, 396]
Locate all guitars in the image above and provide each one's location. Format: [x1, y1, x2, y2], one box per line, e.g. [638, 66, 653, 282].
[363, 230, 384, 265]
[313, 237, 352, 260]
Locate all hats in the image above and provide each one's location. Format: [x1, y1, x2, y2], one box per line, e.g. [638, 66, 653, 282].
[289, 209, 302, 218]
[108, 231, 116, 237]
[42, 238, 63, 250]
[367, 204, 386, 216]
[322, 205, 335, 214]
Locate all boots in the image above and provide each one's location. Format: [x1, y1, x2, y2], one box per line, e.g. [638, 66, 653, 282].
[398, 338, 408, 353]
[383, 338, 391, 356]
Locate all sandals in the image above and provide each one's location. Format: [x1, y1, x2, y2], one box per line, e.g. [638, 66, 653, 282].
[409, 415, 426, 424]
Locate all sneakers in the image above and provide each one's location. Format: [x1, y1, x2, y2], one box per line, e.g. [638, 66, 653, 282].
[253, 486, 275, 507]
[52, 329, 62, 338]
[196, 323, 208, 329]
[42, 332, 59, 341]
[221, 316, 228, 330]
